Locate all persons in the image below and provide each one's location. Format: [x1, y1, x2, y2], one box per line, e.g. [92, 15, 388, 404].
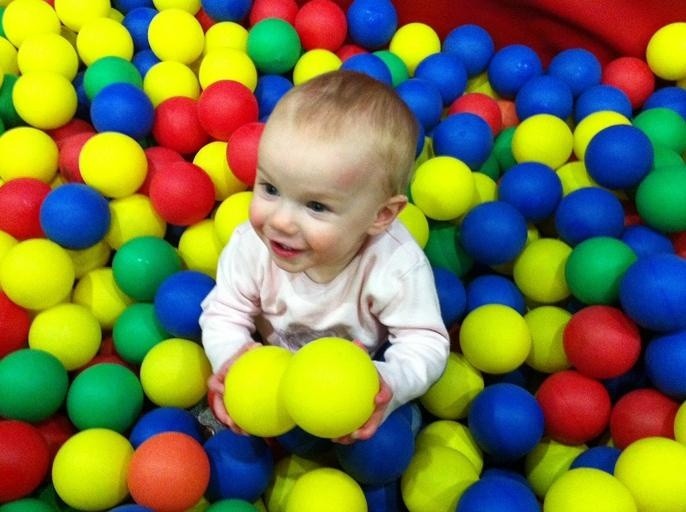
[197, 69, 451, 443]
